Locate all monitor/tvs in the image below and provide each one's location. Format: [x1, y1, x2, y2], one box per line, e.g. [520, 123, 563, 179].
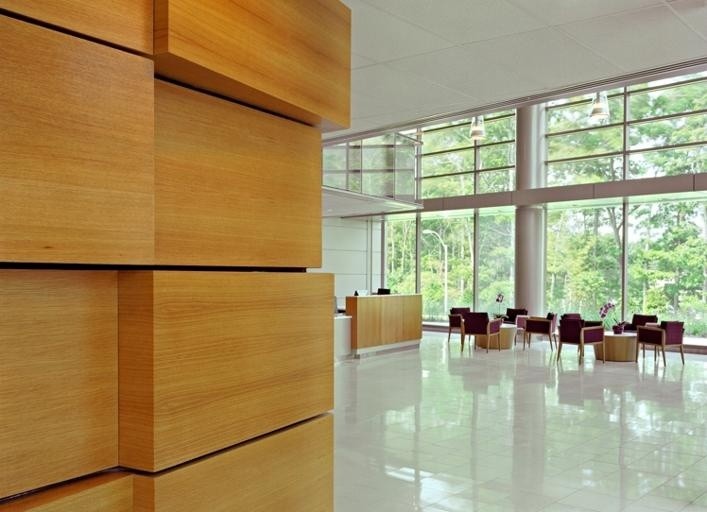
[378, 289, 390, 294]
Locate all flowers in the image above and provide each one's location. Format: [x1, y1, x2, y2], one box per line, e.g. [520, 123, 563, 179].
[492, 292, 510, 319]
[596, 301, 625, 325]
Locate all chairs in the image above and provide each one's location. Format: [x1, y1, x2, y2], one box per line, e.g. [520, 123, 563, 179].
[447, 306, 686, 368]
